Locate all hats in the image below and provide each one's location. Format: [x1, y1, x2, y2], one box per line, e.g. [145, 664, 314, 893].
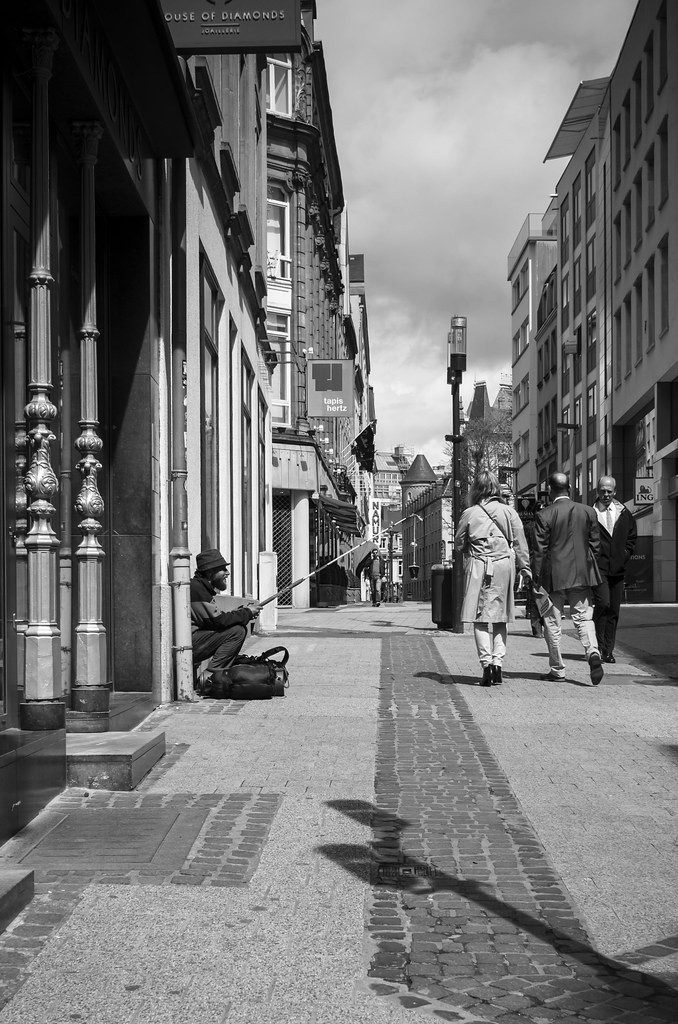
[196, 548, 232, 571]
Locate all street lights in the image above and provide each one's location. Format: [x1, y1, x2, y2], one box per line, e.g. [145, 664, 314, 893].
[445, 316, 468, 636]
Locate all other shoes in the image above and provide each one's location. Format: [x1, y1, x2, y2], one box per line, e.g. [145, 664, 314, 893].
[535, 633, 542, 638]
[602, 653, 615, 663]
[542, 673, 565, 682]
[589, 652, 604, 685]
[376, 603, 380, 607]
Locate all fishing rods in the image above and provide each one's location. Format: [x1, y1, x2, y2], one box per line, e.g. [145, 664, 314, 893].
[259, 513, 424, 610]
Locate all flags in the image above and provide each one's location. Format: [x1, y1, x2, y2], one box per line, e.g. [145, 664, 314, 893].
[351, 425, 374, 473]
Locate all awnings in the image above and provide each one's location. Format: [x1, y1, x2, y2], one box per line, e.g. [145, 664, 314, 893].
[320, 495, 366, 537]
[354, 537, 382, 576]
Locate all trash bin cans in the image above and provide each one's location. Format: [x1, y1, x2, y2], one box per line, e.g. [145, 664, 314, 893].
[430, 564, 453, 629]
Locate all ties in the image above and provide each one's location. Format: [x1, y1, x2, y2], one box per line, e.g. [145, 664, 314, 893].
[606, 508, 613, 537]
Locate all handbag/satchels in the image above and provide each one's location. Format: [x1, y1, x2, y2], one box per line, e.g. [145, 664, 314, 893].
[209, 646, 290, 699]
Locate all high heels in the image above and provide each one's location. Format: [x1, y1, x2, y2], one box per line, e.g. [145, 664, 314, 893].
[480, 664, 492, 686]
[492, 665, 503, 683]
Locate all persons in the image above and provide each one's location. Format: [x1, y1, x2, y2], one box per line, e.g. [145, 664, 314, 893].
[533, 473, 604, 686]
[590, 477, 637, 663]
[454, 472, 533, 688]
[365, 549, 385, 607]
[186, 550, 263, 694]
